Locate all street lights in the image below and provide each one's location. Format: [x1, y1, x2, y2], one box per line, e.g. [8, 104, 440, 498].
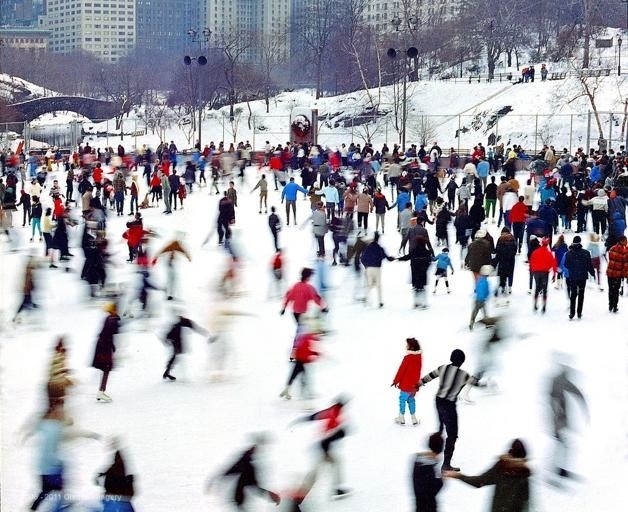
[617, 38, 622, 76]
[184, 26, 212, 150]
[387, 13, 421, 152]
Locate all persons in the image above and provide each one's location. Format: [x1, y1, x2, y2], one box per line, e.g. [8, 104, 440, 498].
[522, 64, 534, 83]
[541, 64, 547, 81]
[0, 141, 628, 510]
